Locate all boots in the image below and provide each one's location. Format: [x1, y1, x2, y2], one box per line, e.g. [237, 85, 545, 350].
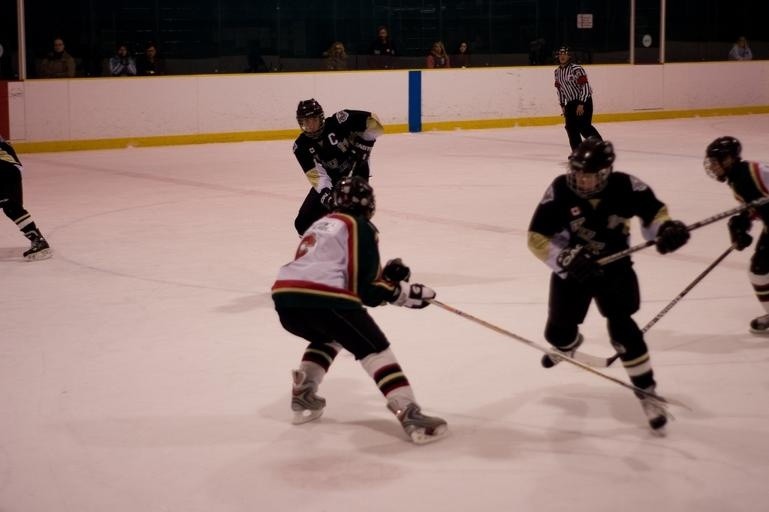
[22, 227, 51, 258]
[633, 380, 667, 431]
[289, 367, 328, 413]
[385, 395, 447, 440]
[540, 333, 585, 368]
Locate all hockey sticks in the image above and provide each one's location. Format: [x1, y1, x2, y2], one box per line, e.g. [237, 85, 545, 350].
[565, 243, 739, 368]
[424, 298, 692, 418]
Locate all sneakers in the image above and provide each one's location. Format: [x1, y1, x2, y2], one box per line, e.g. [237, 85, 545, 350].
[750, 312, 769, 332]
[568, 148, 581, 160]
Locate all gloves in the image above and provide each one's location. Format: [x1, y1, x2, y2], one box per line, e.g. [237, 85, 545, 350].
[655, 219, 692, 256]
[347, 137, 377, 168]
[381, 256, 412, 288]
[389, 280, 437, 311]
[727, 215, 753, 252]
[555, 241, 607, 290]
[317, 186, 333, 213]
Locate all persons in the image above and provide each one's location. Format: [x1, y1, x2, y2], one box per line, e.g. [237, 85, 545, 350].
[0, 136, 53, 262]
[555, 46, 603, 149]
[704, 135, 769, 334]
[527, 135, 691, 436]
[271, 178, 449, 444]
[291, 99, 386, 238]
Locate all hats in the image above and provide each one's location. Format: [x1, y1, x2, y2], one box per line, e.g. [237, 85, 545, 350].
[554, 46, 573, 59]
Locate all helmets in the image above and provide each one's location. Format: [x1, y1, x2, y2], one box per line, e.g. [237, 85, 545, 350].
[566, 136, 618, 196]
[702, 135, 742, 183]
[331, 177, 377, 221]
[294, 98, 327, 140]
